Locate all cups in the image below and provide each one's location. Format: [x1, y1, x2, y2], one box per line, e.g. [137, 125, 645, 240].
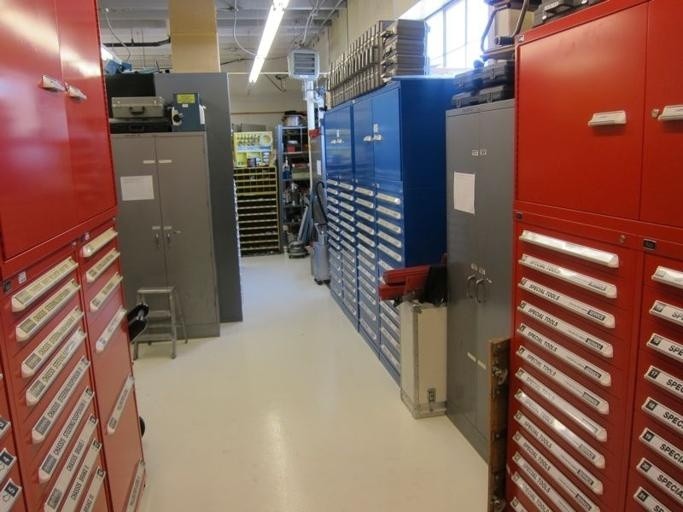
[247, 158, 256, 167]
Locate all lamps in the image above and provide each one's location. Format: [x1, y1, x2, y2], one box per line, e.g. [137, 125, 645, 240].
[247, 1, 291, 85]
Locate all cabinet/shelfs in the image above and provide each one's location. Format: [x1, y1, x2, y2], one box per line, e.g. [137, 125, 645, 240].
[447, 104, 515, 462]
[513, 2, 683, 509]
[279, 128, 311, 249]
[325, 84, 406, 384]
[236, 164, 281, 254]
[0, 0, 144, 512]
[109, 131, 220, 343]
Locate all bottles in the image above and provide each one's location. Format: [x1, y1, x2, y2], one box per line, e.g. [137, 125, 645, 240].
[282, 160, 291, 179]
[263, 152, 270, 167]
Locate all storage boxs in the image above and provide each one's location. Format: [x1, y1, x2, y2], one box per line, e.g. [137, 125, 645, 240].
[308, 225, 329, 287]
[397, 295, 445, 423]
[450, 63, 512, 108]
[325, 17, 429, 108]
